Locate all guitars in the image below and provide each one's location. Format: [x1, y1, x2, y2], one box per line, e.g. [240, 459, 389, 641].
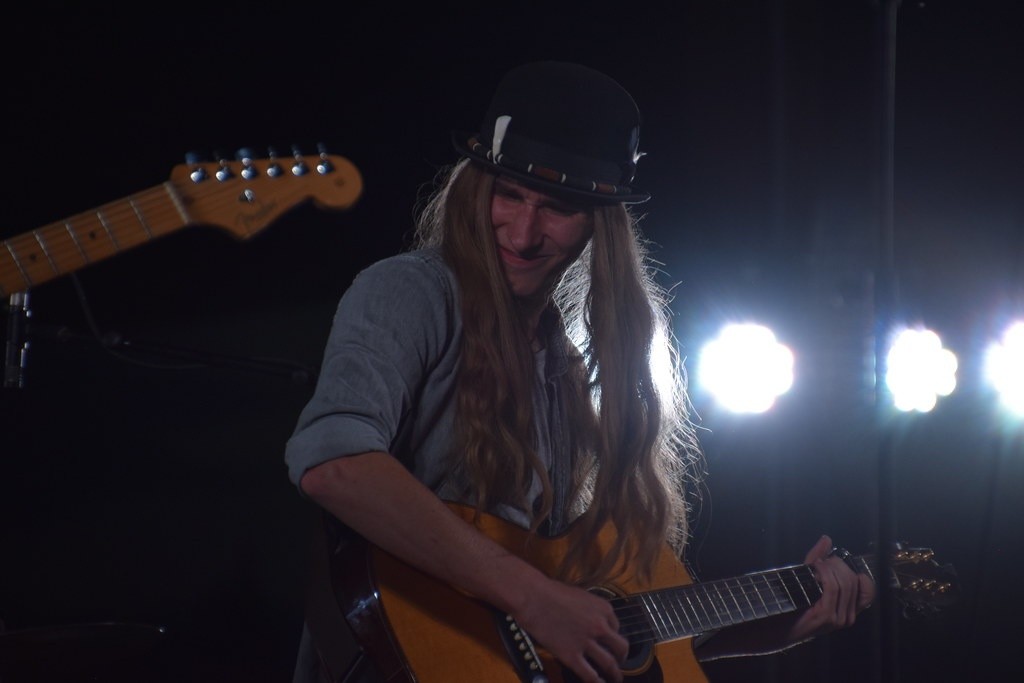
[0, 143, 367, 305]
[305, 483, 961, 683]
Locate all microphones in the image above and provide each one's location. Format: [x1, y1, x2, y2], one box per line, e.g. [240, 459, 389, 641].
[4, 290, 33, 390]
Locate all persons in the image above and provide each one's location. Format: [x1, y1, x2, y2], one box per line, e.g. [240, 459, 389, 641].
[283, 62, 876, 683]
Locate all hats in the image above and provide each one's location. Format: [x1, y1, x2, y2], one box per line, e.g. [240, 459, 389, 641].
[453, 60, 655, 204]
